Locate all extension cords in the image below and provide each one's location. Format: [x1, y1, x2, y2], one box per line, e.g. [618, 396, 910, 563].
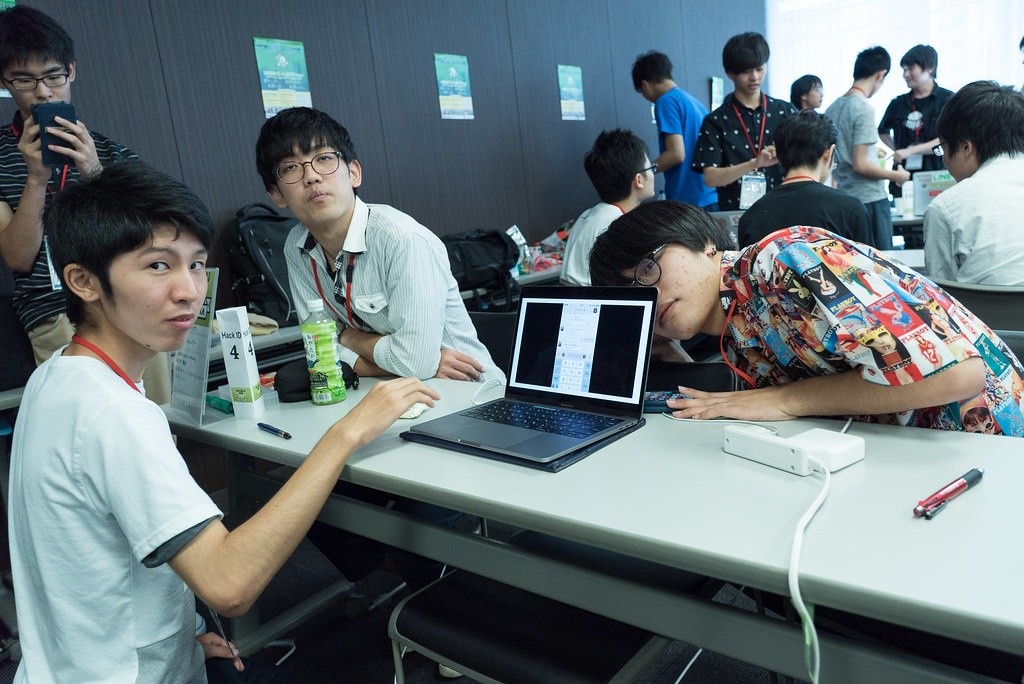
[722, 425, 814, 476]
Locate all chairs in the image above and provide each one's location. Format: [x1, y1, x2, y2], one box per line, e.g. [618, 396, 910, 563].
[927, 276, 1024, 331]
[388, 530, 724, 684]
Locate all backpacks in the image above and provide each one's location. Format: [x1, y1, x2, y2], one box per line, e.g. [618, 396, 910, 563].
[217, 202, 306, 328]
[440, 229, 520, 312]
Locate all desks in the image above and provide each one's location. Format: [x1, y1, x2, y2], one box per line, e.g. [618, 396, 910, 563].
[159, 376, 1024, 684]
[891, 208, 924, 250]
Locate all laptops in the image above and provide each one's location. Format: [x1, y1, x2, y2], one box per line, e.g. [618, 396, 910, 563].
[707, 210, 746, 252]
[411, 285, 662, 463]
[914, 170, 958, 217]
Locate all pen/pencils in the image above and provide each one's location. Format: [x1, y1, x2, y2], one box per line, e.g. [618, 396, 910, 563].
[257, 421, 291, 439]
[913, 466, 984, 519]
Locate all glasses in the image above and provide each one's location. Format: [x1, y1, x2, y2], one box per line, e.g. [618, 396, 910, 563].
[631, 162, 660, 183]
[0, 62, 70, 91]
[269, 151, 345, 185]
[630, 243, 677, 288]
[931, 141, 954, 157]
[831, 149, 841, 170]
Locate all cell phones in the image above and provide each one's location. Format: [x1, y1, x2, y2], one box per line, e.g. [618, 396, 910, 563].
[30, 99, 78, 169]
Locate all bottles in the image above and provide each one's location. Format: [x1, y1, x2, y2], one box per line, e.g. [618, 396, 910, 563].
[726, 231, 739, 251]
[534, 242, 542, 261]
[657, 190, 666, 200]
[879, 151, 885, 169]
[300, 299, 347, 405]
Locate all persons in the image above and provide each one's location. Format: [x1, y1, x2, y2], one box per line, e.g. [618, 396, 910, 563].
[255, 107, 508, 385]
[7, 163, 441, 684]
[0, 6, 142, 450]
[559, 32, 1024, 293]
[588, 200, 1024, 440]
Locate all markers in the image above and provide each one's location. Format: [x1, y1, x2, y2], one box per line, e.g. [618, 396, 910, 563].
[205, 394, 234, 413]
